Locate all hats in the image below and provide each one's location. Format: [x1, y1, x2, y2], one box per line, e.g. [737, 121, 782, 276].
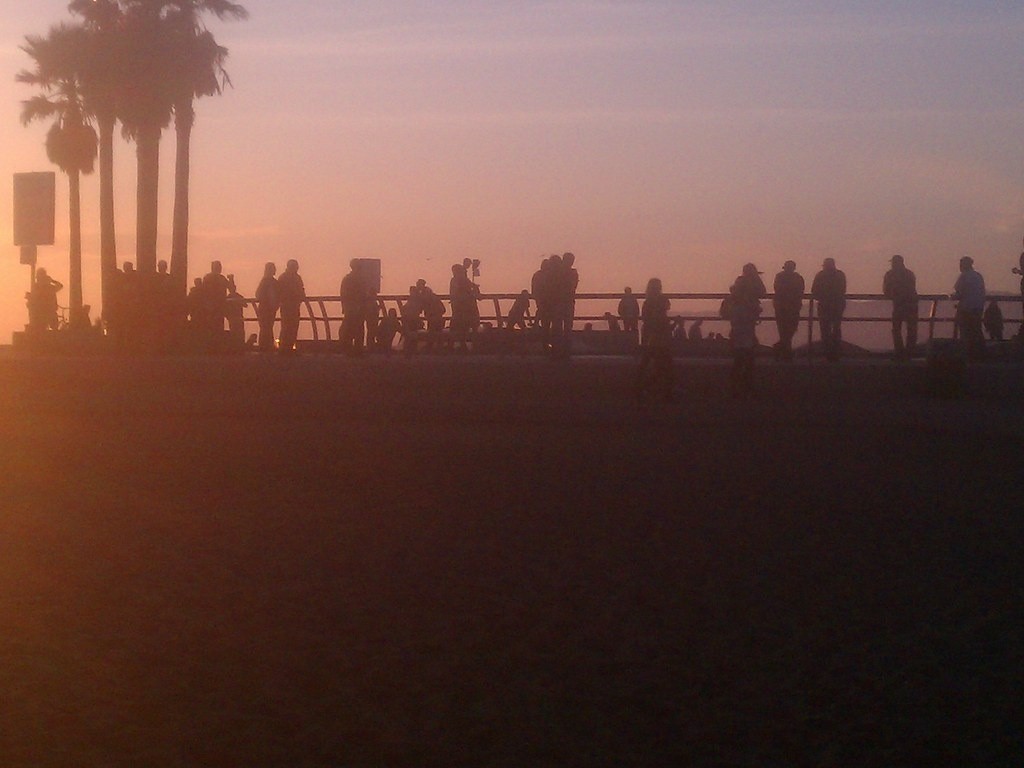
[888, 255, 904, 263]
[781, 260, 797, 269]
[743, 263, 764, 274]
[821, 258, 835, 267]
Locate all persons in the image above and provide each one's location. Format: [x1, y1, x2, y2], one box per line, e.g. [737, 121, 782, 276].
[35, 268, 63, 329]
[771, 260, 805, 352]
[619, 287, 639, 330]
[810, 258, 847, 341]
[954, 256, 987, 349]
[335, 258, 483, 354]
[97, 261, 248, 347]
[641, 261, 767, 348]
[1013, 252, 1023, 311]
[604, 311, 620, 332]
[276, 259, 306, 352]
[881, 254, 919, 357]
[252, 262, 279, 352]
[508, 252, 579, 347]
[983, 300, 1004, 340]
